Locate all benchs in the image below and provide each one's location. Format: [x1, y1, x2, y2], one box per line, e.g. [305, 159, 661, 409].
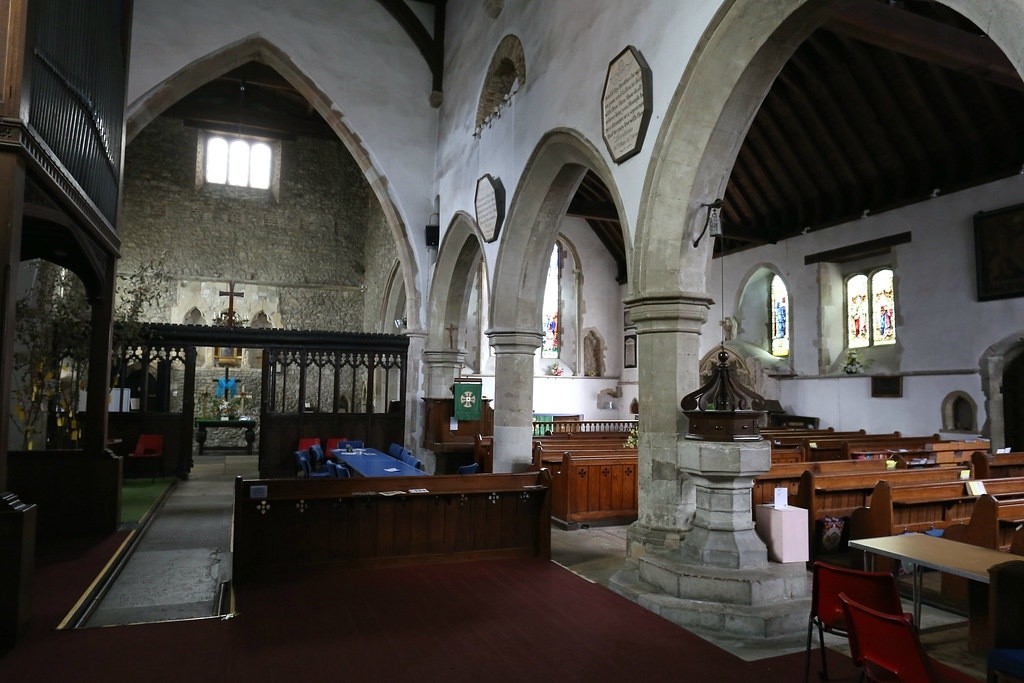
[474, 428, 1024, 659]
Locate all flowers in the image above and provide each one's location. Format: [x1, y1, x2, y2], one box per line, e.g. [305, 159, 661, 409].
[838, 350, 864, 375]
[622, 429, 639, 449]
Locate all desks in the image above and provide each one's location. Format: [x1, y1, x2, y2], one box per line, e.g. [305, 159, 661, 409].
[847, 532, 1024, 634]
[107, 438, 122, 444]
[329, 448, 431, 477]
[195, 416, 256, 455]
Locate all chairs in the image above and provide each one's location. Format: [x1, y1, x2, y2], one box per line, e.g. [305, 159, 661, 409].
[294, 437, 364, 478]
[389, 443, 478, 475]
[986, 560, 1024, 683]
[802, 562, 936, 683]
[123, 434, 164, 484]
